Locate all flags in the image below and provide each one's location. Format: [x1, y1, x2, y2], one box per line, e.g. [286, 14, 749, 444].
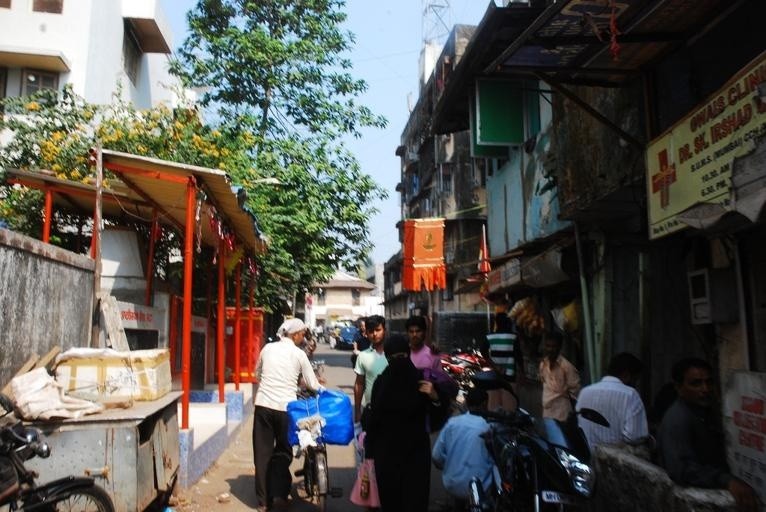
[478, 225, 491, 274]
[401, 218, 446, 292]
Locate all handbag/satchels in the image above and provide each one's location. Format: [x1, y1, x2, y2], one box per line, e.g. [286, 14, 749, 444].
[349, 432, 383, 510]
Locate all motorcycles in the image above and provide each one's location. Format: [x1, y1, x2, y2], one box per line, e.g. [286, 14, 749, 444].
[439, 338, 488, 385]
[468, 380, 611, 512]
[0, 394, 116, 511]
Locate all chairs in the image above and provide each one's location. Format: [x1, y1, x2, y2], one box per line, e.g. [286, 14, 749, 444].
[587, 437, 762, 512]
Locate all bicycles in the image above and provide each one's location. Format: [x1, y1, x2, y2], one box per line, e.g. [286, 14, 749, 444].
[295, 358, 344, 510]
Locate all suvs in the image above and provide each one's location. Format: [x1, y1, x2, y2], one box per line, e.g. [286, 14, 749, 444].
[432, 308, 495, 353]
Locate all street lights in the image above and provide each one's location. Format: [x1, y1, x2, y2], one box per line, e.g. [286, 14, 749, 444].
[245, 177, 282, 186]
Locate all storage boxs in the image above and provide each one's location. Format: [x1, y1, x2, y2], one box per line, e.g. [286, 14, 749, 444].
[55, 358, 136, 408]
[128, 352, 172, 400]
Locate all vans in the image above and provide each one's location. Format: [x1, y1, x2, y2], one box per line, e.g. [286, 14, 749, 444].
[381, 317, 408, 346]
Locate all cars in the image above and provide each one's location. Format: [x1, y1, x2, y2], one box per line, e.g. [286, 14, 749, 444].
[337, 328, 361, 349]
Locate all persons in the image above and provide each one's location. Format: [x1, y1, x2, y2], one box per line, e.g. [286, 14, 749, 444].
[251, 312, 765, 510]
